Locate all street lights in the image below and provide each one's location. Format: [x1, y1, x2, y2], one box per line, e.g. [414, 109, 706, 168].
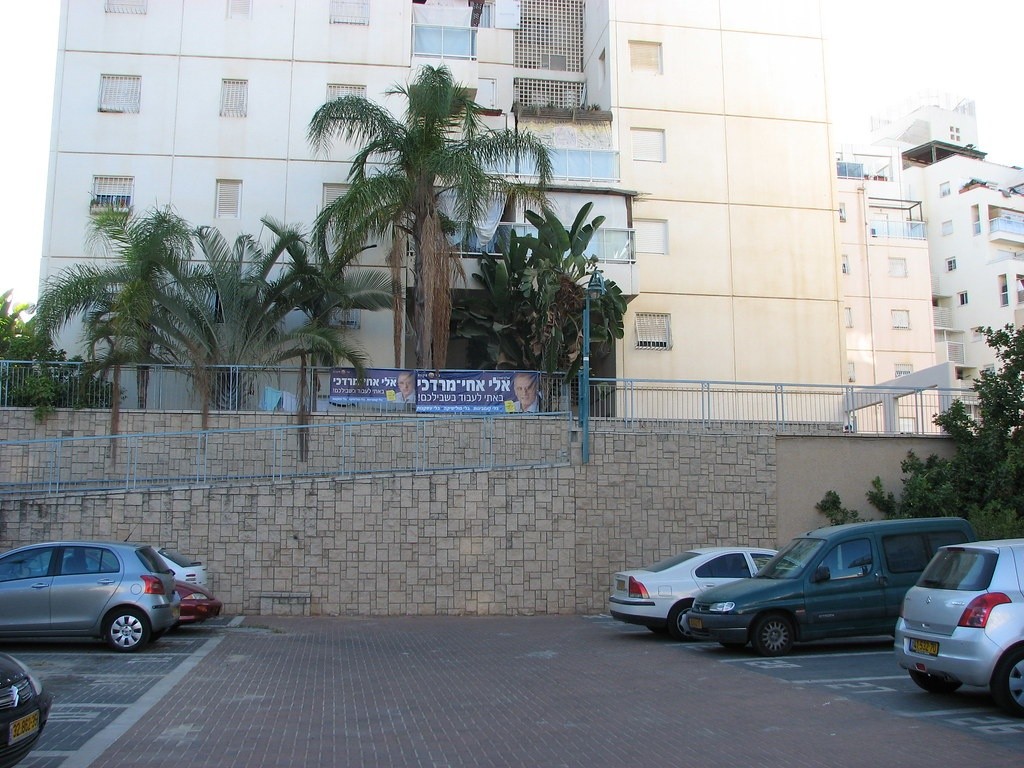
[578, 270, 607, 464]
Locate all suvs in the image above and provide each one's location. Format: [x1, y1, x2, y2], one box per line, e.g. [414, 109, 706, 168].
[894, 535, 1024, 718]
[687, 516, 980, 659]
[0, 540, 183, 653]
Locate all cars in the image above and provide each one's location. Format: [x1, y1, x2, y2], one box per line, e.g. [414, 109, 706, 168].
[0, 649, 55, 768]
[609, 545, 807, 643]
[22, 544, 224, 633]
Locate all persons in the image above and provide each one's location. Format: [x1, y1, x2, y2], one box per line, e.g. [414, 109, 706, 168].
[393, 371, 416, 402]
[512, 373, 539, 412]
